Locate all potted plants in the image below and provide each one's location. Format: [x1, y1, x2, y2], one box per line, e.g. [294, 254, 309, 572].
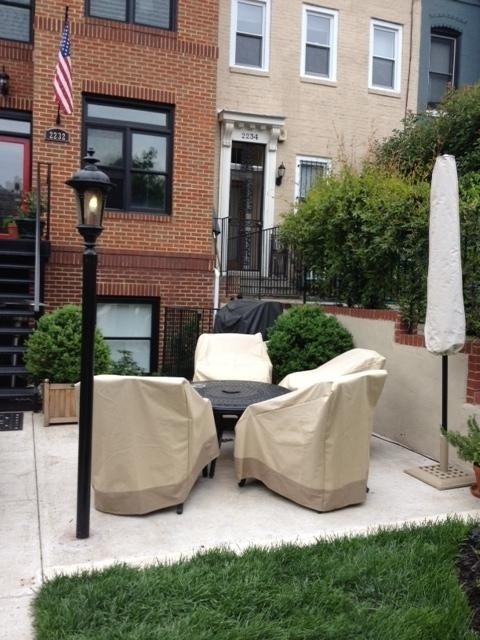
[21, 303, 110, 427]
[437, 412, 480, 497]
[14, 192, 48, 239]
[3, 217, 17, 236]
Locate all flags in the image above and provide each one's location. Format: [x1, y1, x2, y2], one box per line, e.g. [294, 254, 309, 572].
[53, 18, 76, 117]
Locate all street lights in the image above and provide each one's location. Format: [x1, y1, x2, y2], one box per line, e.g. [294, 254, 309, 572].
[60, 142, 118, 544]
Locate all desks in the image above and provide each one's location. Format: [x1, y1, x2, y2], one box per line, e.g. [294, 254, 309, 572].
[187, 379, 289, 478]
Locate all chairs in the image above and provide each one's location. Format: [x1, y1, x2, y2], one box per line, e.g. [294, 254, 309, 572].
[90, 374, 220, 516]
[192, 331, 273, 383]
[278, 347, 387, 392]
[234, 370, 390, 513]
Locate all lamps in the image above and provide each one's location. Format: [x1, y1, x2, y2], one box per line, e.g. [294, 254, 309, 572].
[275, 162, 288, 186]
[1, 63, 9, 99]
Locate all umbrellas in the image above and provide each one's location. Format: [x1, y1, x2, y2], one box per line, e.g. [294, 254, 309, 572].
[404, 150, 478, 489]
